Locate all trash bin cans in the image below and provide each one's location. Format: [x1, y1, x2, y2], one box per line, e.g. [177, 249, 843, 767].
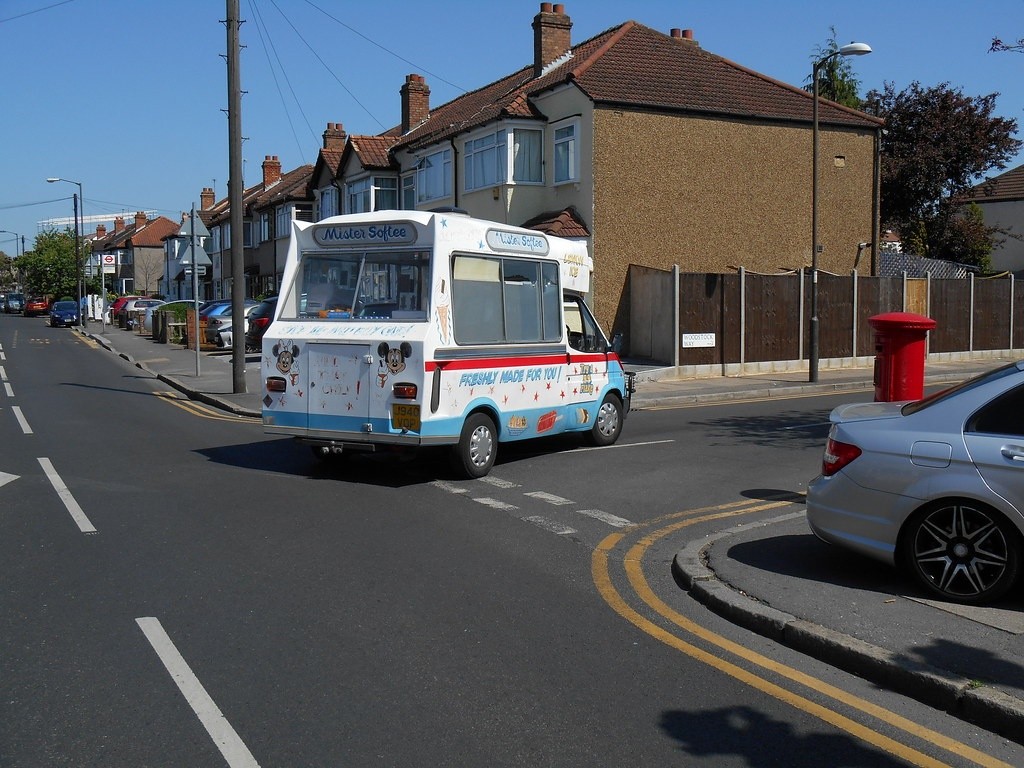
[119, 309, 127, 328]
[127, 310, 139, 331]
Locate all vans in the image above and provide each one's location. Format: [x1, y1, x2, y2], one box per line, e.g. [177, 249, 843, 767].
[261, 209, 636, 478]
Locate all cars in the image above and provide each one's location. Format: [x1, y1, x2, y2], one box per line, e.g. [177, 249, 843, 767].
[49, 301, 85, 327]
[24, 296, 49, 316]
[805, 357, 1023, 605]
[0, 292, 26, 314]
[110, 294, 308, 354]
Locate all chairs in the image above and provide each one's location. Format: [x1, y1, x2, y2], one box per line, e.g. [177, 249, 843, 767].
[392, 311, 427, 319]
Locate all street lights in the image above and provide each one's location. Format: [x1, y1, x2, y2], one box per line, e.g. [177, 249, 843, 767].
[809, 42, 872, 383]
[0, 230, 20, 292]
[46, 178, 88, 328]
